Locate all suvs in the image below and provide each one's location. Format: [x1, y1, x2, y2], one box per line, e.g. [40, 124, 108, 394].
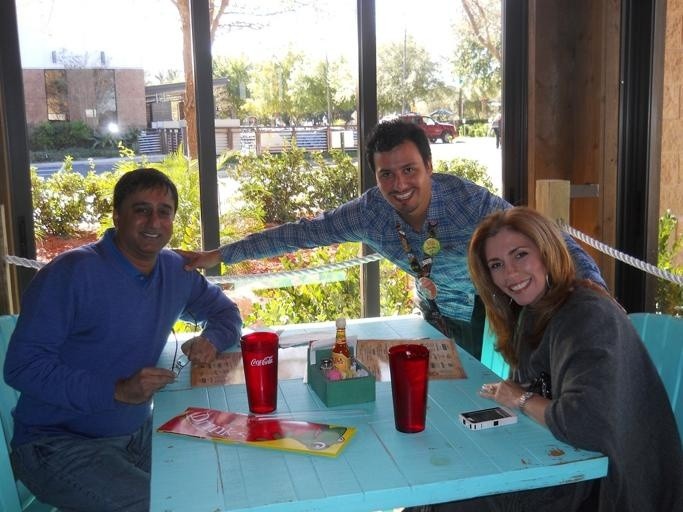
[397, 113, 458, 146]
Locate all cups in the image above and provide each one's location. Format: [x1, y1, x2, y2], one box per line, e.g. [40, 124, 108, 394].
[387, 343, 429, 433]
[240, 331, 280, 414]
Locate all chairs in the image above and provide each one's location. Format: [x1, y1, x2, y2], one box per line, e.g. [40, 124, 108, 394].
[480, 314, 512, 382]
[0, 314, 60, 512]
[626, 312, 683, 448]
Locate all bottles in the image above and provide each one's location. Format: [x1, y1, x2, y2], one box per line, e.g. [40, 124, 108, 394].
[331, 315, 351, 372]
[321, 358, 357, 376]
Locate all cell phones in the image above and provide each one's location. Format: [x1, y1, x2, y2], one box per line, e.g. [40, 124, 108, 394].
[459, 406, 517, 431]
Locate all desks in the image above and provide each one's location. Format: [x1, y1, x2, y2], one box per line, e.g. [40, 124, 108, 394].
[148, 314, 609, 512]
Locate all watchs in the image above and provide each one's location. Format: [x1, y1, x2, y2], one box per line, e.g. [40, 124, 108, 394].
[518, 390, 541, 416]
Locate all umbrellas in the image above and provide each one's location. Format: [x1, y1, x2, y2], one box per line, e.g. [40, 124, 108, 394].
[427, 108, 454, 118]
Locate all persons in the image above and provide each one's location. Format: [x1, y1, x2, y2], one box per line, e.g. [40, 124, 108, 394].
[0, 166, 242, 512]
[465, 207, 682, 511]
[491, 109, 501, 149]
[161, 119, 632, 376]
